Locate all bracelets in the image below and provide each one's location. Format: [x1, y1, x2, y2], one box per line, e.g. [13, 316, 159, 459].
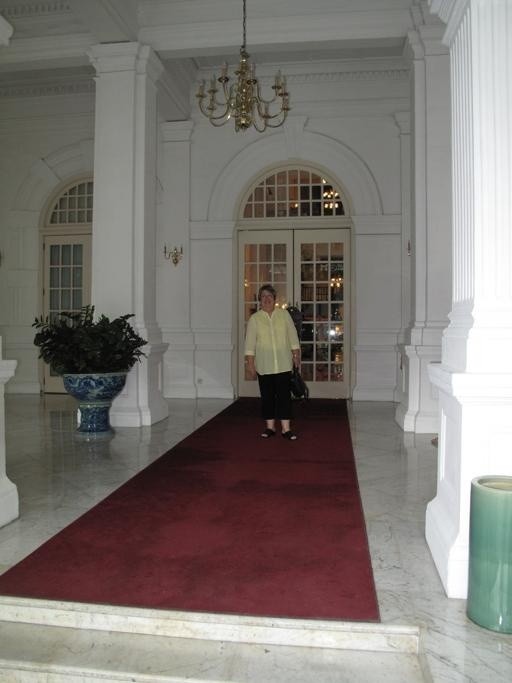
[293, 353, 300, 359]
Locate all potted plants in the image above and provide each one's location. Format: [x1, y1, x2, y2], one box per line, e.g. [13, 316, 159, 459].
[28, 299, 150, 445]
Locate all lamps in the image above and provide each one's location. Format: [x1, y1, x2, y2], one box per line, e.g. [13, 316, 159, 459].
[195, 0, 295, 136]
[160, 240, 186, 267]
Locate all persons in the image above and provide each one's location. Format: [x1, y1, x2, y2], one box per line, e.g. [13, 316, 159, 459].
[243, 284, 301, 441]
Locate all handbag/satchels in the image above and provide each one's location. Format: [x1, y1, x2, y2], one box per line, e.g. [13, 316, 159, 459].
[289, 365, 306, 399]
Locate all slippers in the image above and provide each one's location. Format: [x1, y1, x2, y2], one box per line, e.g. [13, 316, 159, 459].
[260, 428, 277, 438]
[282, 430, 297, 440]
